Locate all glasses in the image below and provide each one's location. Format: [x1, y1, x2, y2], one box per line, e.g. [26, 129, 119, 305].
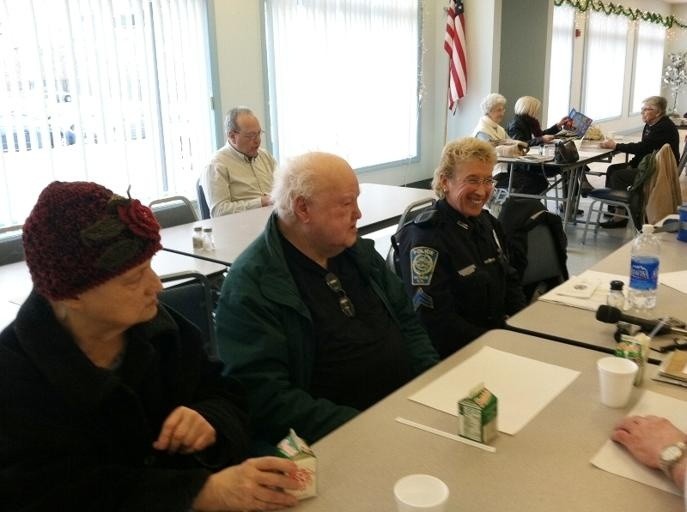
[231, 128, 265, 140]
[452, 177, 497, 187]
[324, 270, 357, 318]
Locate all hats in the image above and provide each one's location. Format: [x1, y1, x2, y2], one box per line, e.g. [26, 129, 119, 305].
[18, 177, 165, 301]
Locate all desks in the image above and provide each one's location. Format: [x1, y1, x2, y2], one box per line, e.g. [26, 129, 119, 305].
[506, 213, 686, 360]
[288, 329, 684, 511]
[495, 136, 629, 233]
[0, 182, 439, 330]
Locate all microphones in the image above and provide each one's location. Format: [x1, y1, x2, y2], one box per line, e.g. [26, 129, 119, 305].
[593, 302, 671, 335]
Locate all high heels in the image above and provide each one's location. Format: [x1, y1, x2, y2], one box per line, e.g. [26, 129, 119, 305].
[580, 187, 595, 198]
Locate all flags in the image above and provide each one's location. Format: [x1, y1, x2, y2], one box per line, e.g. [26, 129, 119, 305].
[443, 0, 469, 115]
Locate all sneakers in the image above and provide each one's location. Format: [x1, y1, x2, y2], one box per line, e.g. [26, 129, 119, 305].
[599, 216, 629, 229]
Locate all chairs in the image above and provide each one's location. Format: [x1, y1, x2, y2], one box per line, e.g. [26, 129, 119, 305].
[579, 187, 636, 245]
[675, 144, 687, 178]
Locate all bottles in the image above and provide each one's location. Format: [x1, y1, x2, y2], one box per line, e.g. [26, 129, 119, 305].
[191, 226, 215, 252]
[538, 144, 549, 157]
[627, 223, 659, 308]
[607, 281, 626, 312]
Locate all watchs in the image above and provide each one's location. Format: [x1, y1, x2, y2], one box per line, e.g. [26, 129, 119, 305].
[659, 438, 687, 480]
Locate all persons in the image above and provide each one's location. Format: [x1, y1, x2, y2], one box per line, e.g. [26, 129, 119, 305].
[609, 414, 687, 495]
[390, 135, 532, 360]
[0, 182, 301, 512]
[510, 90, 596, 216]
[212, 150, 439, 442]
[598, 95, 682, 229]
[470, 94, 549, 206]
[203, 107, 279, 218]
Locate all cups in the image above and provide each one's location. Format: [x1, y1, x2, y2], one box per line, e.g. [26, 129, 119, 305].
[391, 474, 449, 511]
[494, 144, 512, 158]
[596, 356, 637, 409]
[676, 204, 686, 241]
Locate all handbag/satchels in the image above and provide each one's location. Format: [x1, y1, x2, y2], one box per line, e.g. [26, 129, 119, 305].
[553, 139, 580, 165]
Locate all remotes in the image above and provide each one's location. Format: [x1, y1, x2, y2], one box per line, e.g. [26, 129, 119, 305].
[614, 315, 687, 343]
